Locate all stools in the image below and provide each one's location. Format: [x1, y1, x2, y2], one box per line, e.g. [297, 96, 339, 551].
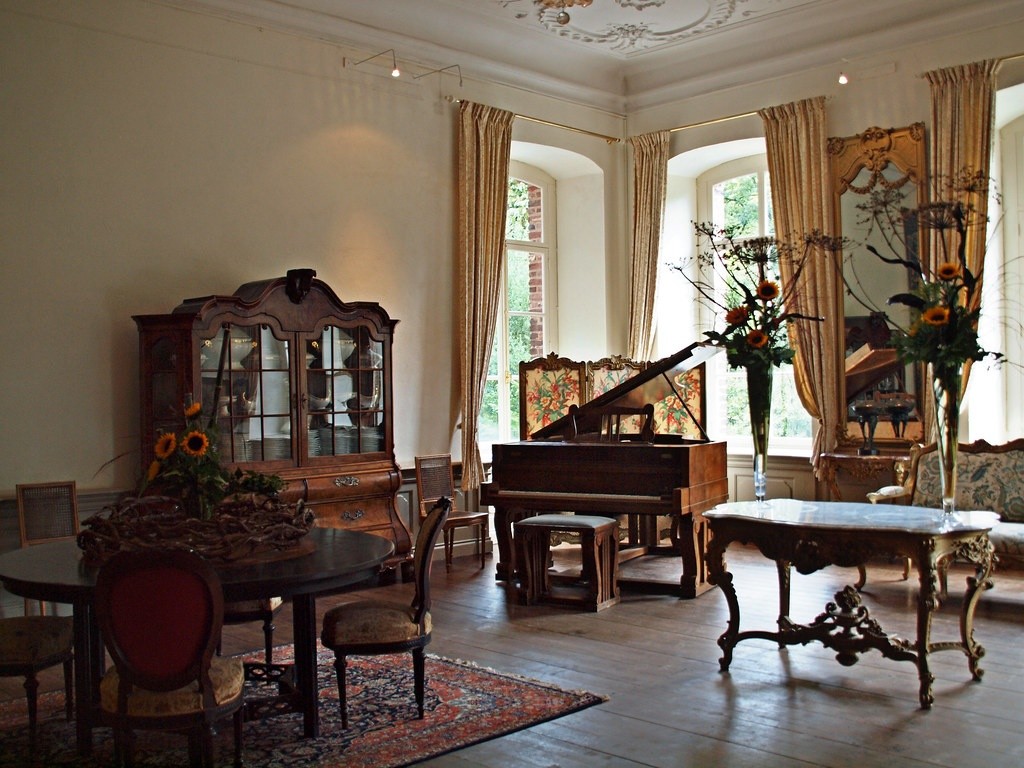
[514, 515, 621, 612]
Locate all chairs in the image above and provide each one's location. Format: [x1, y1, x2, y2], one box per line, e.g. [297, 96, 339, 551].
[0, 453, 490, 768]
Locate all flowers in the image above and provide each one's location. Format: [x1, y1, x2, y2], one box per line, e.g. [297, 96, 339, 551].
[666, 172, 1024, 492]
[93, 391, 292, 521]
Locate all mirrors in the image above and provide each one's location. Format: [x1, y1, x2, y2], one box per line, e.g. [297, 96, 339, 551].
[828, 120, 930, 444]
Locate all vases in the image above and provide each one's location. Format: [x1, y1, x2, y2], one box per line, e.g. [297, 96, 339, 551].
[76, 497, 316, 574]
[747, 365, 772, 510]
[931, 364, 962, 523]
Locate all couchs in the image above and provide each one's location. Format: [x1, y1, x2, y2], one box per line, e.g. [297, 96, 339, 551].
[854, 439, 1024, 602]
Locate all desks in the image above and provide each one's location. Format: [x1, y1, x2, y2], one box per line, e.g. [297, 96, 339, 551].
[702, 499, 1002, 709]
[0, 527, 396, 757]
[819, 450, 919, 503]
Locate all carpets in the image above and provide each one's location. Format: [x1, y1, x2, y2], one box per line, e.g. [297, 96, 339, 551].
[0, 635, 612, 768]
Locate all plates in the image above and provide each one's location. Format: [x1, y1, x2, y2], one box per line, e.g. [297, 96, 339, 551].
[218, 424, 384, 464]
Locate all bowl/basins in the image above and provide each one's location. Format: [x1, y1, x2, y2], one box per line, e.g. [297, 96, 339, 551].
[213, 336, 252, 369]
[237, 392, 257, 414]
[308, 393, 330, 409]
[306, 352, 316, 368]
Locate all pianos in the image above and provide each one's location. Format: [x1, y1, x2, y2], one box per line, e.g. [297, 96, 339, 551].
[478, 340, 731, 599]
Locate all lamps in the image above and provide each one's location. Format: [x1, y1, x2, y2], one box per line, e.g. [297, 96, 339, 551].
[355, 49, 399, 77]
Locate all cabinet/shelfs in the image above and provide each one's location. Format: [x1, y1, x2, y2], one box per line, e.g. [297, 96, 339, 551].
[130, 268, 413, 619]
